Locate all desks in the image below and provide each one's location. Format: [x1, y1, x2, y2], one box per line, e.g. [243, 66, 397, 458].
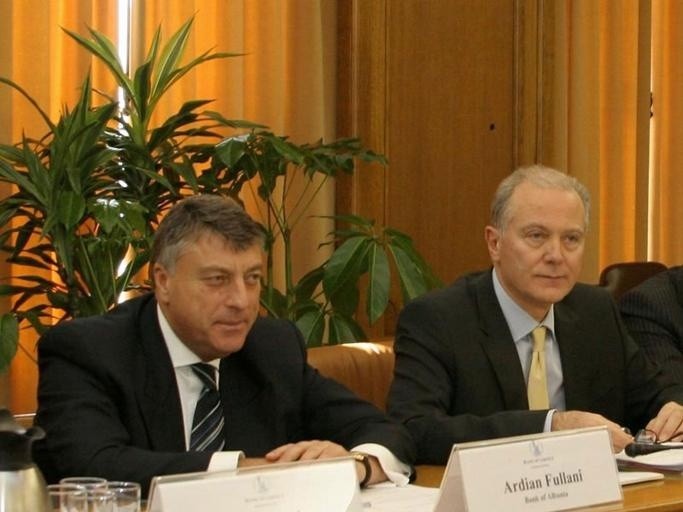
[411, 465, 683, 512]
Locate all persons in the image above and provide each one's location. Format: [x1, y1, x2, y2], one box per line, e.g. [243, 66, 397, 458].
[378, 163, 682, 462]
[620, 262, 682, 400]
[32, 190, 420, 491]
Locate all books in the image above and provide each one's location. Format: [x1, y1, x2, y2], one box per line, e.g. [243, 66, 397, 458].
[619, 471, 665, 489]
[612, 441, 682, 473]
[359, 485, 441, 512]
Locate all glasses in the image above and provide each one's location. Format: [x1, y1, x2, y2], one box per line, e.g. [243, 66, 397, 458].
[620, 426, 683, 445]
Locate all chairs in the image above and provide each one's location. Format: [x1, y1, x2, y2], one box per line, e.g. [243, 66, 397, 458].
[590, 261, 666, 299]
[297, 340, 397, 415]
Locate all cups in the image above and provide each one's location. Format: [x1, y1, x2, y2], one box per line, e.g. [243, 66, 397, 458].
[47, 475, 143, 512]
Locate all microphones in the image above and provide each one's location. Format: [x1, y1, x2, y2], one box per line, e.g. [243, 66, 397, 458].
[626, 442, 683, 458]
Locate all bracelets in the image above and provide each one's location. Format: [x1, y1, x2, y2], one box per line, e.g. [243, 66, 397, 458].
[352, 453, 373, 487]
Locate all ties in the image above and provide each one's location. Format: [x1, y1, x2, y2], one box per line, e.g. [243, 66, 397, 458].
[525, 325, 552, 411]
[186, 361, 229, 453]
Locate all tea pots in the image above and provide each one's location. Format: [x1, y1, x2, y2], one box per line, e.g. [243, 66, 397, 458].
[0, 406, 53, 512]
